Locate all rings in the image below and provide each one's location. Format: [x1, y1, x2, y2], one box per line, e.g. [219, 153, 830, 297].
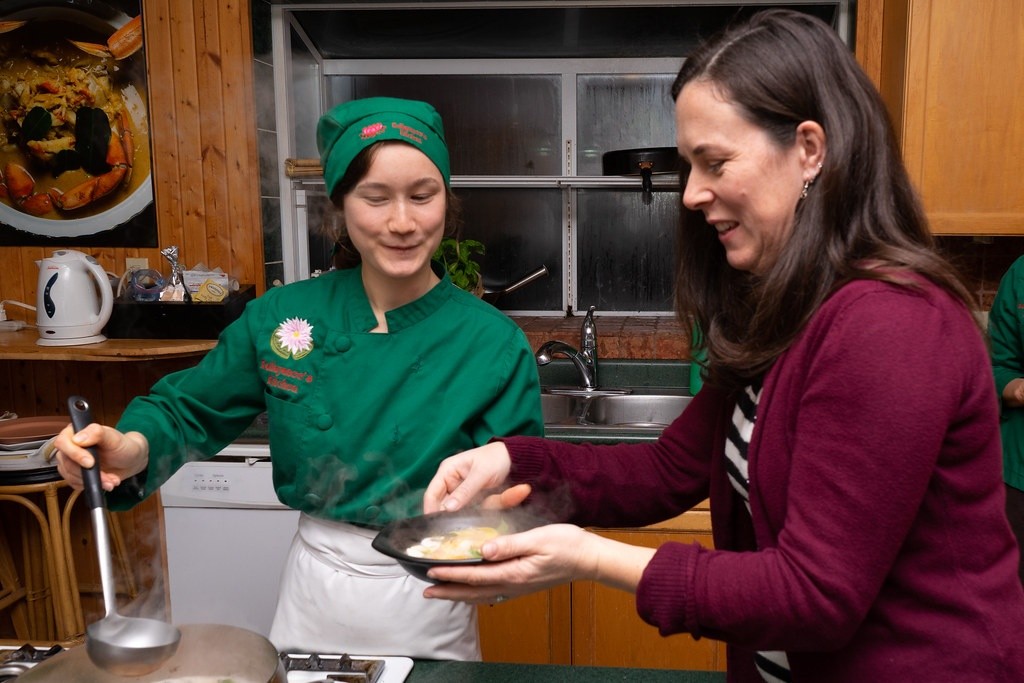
[495, 595, 508, 604]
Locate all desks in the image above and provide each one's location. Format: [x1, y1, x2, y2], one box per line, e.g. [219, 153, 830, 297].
[0, 323, 221, 362]
[401, 659, 731, 683]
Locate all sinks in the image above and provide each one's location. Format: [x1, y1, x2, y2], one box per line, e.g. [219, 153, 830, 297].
[537, 389, 697, 441]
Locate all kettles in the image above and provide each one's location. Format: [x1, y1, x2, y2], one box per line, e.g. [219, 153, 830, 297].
[34, 250, 114, 346]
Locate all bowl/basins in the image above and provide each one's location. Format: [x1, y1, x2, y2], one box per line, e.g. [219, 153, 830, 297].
[372, 509, 555, 584]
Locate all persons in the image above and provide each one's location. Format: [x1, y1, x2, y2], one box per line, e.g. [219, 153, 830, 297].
[52, 97, 546, 665]
[984, 252, 1024, 587]
[420, 6, 1024, 683]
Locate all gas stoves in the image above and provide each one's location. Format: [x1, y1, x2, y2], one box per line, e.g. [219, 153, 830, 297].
[279, 652, 415, 683]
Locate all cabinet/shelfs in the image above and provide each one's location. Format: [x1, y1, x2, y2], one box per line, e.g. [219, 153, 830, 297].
[853, 0, 1024, 237]
[474, 510, 728, 673]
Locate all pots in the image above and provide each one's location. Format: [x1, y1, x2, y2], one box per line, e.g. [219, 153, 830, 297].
[602, 147, 679, 195]
[1, 622, 289, 683]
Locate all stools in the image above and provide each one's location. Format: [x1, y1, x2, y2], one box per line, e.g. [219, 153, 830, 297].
[0, 465, 138, 643]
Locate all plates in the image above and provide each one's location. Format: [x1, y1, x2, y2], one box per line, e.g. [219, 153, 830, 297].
[0, 416, 72, 451]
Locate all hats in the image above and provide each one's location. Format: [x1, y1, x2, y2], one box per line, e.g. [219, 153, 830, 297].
[316, 97, 451, 199]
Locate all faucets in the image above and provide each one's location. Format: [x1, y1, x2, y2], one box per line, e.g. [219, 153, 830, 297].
[534, 304, 600, 392]
